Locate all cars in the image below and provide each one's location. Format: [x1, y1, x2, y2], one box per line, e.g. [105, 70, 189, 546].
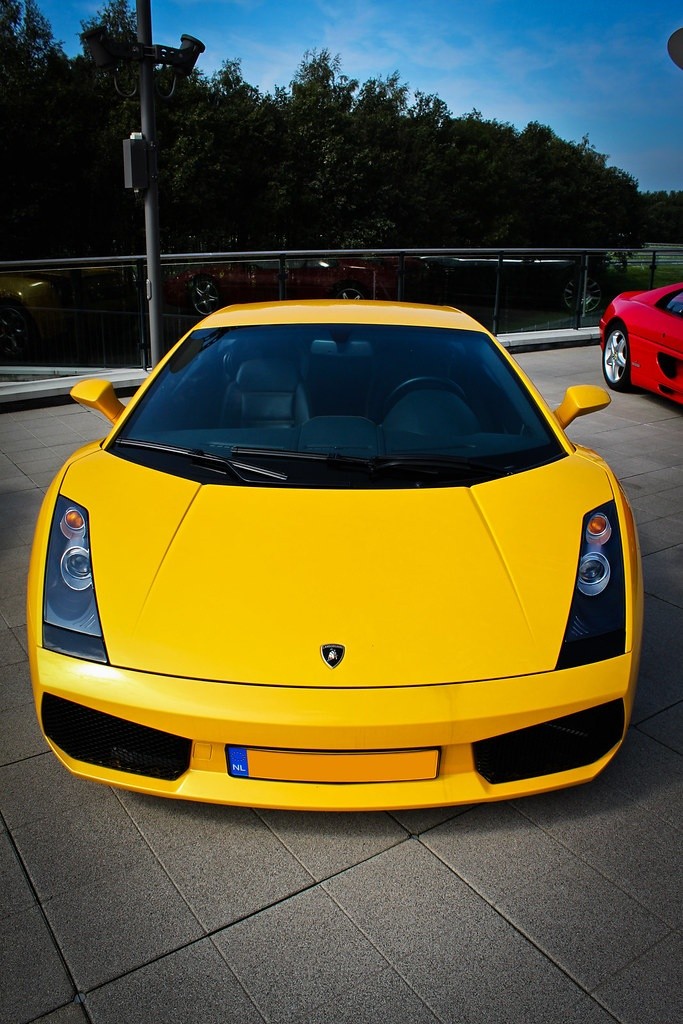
[0, 251, 629, 364]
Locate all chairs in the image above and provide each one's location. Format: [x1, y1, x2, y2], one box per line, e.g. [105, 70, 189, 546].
[215, 340, 312, 429]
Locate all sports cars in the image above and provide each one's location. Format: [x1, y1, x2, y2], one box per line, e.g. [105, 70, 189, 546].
[598, 283, 683, 404]
[26, 296, 645, 814]
